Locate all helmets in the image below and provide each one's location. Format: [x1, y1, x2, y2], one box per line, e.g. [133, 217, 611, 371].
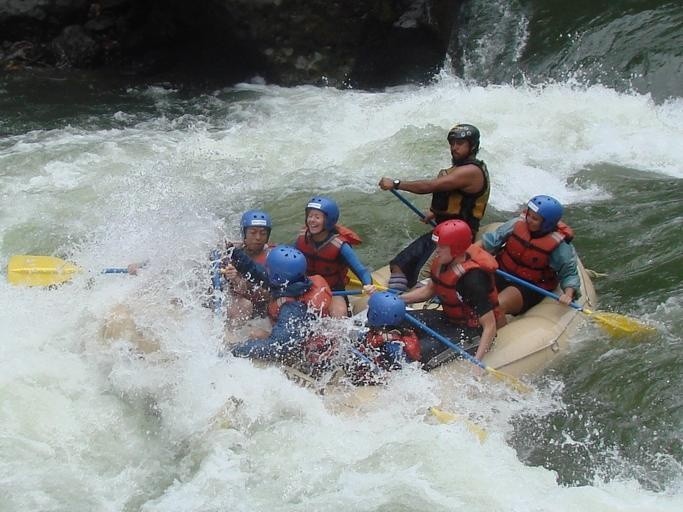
[240, 209, 272, 242]
[365, 290, 406, 327]
[305, 197, 340, 233]
[431, 218, 473, 257]
[525, 195, 564, 237]
[446, 122, 479, 164]
[265, 244, 307, 287]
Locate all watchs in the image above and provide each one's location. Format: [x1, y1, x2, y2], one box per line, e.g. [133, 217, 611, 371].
[392, 178, 401, 191]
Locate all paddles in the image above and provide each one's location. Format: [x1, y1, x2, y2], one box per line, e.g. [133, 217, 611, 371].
[496, 267, 655, 335]
[8, 257, 228, 286]
[405, 311, 545, 402]
[346, 268, 441, 303]
[348, 343, 488, 442]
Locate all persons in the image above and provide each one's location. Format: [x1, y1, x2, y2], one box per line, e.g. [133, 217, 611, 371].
[472, 194, 581, 330]
[126, 193, 424, 386]
[362, 218, 509, 369]
[376, 123, 491, 289]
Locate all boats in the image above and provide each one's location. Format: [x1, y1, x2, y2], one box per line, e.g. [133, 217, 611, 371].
[102, 219, 598, 474]
